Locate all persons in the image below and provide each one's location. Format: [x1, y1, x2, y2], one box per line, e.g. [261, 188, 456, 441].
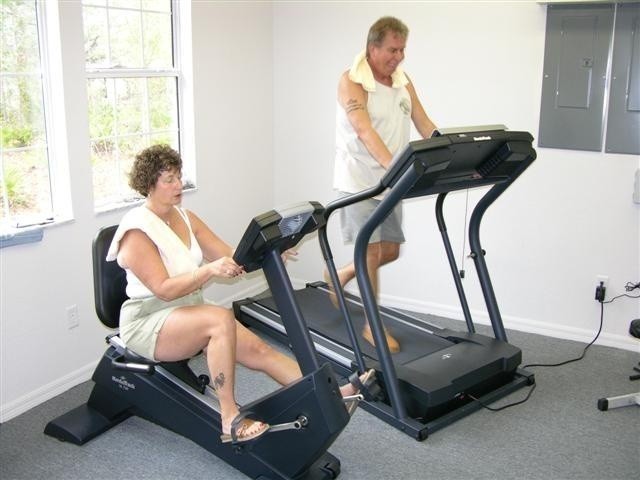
[106, 143, 376, 446]
[323, 15, 438, 356]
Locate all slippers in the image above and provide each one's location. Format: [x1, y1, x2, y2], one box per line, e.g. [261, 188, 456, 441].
[344, 369, 377, 414]
[220, 417, 270, 443]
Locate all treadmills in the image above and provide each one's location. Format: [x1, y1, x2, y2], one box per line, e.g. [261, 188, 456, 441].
[231, 122, 537, 442]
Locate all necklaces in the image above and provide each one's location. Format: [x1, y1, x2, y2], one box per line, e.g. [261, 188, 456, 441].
[145, 203, 173, 227]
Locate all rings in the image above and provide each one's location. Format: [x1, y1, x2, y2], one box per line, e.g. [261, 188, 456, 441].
[226, 268, 231, 275]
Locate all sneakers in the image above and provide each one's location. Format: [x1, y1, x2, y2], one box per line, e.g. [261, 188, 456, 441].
[324, 268, 346, 309]
[361, 326, 401, 355]
